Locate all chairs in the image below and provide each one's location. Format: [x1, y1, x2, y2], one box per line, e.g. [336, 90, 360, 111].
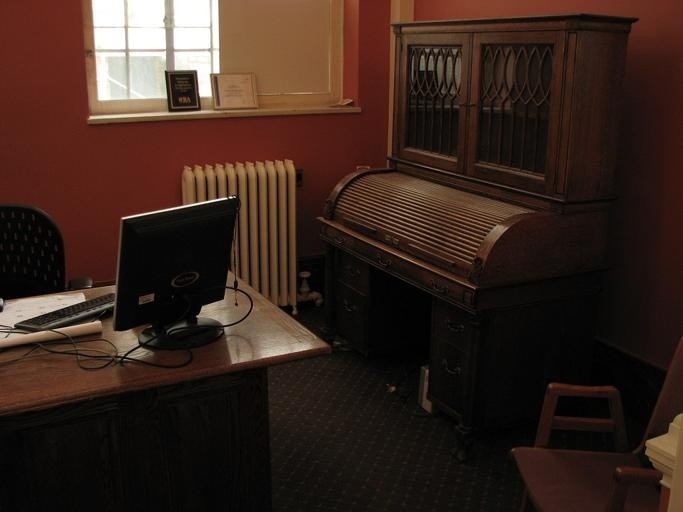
[509, 335, 683, 512]
[0, 202, 65, 301]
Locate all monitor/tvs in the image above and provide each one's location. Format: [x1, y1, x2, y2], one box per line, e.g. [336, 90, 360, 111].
[113, 197, 239, 350]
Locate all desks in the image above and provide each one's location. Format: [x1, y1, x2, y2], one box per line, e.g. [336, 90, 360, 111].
[0, 271, 332, 512]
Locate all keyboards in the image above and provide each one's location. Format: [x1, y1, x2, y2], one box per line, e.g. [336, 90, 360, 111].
[14, 294, 115, 330]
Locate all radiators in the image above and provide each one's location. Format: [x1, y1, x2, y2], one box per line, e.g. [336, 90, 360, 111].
[180, 160, 323, 316]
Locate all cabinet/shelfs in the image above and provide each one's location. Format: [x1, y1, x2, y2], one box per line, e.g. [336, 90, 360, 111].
[386, 13, 638, 214]
[424, 298, 598, 433]
[329, 246, 428, 346]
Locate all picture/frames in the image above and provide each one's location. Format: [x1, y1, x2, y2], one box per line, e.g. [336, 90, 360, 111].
[210, 72, 259, 110]
[164, 71, 201, 111]
